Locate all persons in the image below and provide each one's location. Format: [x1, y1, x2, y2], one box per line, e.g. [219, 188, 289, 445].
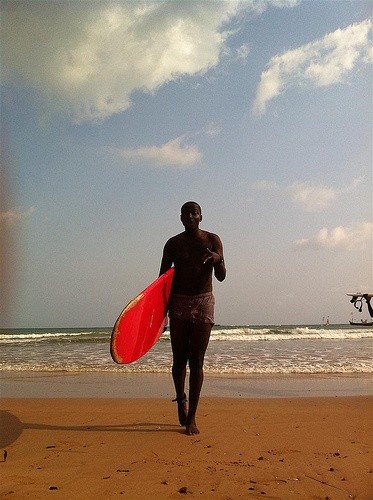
[157, 201, 226, 435]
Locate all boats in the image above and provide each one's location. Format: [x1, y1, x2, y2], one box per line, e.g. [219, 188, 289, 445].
[349, 320, 373, 326]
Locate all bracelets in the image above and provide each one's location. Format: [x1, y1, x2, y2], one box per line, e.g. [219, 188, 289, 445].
[217, 255, 224, 266]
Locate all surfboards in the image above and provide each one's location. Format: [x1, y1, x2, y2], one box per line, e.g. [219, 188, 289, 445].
[111, 267, 173, 365]
